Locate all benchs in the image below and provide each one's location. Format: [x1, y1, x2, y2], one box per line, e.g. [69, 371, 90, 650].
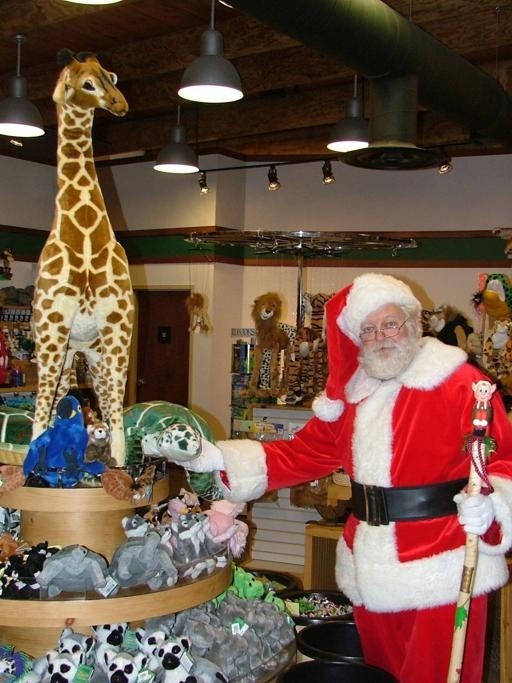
[302, 519, 511, 682]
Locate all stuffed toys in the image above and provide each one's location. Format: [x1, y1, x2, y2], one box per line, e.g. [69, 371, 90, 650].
[28, 49, 136, 468]
[0, 53, 333, 683]
[0, 395, 295, 683]
[244, 291, 331, 408]
[421, 273, 512, 414]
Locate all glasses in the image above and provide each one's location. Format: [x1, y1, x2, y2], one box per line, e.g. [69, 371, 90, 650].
[360, 316, 410, 341]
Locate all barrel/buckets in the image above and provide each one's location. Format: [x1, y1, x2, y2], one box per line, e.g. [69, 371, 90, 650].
[243, 568, 397, 683]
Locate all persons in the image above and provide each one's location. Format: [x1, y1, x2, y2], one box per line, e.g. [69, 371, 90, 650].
[170, 272, 512, 683]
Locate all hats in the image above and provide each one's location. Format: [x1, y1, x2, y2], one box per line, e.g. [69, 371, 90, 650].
[312, 274, 422, 422]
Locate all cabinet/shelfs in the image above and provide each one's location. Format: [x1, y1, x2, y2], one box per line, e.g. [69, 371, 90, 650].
[231, 405, 327, 590]
[0, 472, 299, 682]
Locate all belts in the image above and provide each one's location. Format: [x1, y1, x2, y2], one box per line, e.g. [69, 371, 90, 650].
[348, 478, 468, 525]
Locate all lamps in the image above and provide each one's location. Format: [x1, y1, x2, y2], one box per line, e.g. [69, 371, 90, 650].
[439, 151, 454, 177]
[318, 160, 337, 186]
[198, 171, 210, 194]
[326, 70, 372, 153]
[174, 0, 247, 104]
[6, 136, 23, 149]
[2, 35, 46, 138]
[266, 166, 282, 193]
[151, 103, 201, 175]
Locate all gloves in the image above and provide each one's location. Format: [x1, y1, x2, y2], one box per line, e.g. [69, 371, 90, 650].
[168, 438, 225, 472]
[453, 492, 495, 534]
